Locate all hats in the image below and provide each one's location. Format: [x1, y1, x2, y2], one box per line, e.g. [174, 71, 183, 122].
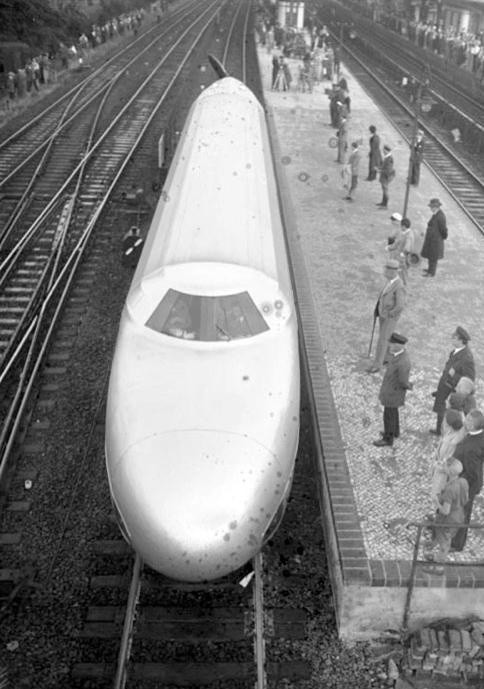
[383, 259, 401, 272]
[456, 326, 471, 340]
[428, 199, 441, 207]
[392, 212, 402, 220]
[387, 333, 408, 344]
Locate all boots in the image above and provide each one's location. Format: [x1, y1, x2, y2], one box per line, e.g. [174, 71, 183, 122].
[376, 196, 388, 209]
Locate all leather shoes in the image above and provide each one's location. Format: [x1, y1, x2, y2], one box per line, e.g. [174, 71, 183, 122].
[423, 268, 434, 276]
[373, 430, 397, 447]
[366, 367, 379, 372]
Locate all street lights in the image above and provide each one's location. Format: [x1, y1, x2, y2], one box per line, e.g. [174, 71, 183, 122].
[328, 19, 359, 84]
[393, 76, 439, 225]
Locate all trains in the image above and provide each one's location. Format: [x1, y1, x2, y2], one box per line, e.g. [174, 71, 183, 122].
[100, 71, 307, 589]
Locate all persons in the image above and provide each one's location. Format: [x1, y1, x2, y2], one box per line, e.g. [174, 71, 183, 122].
[386, 237, 407, 286]
[345, 141, 361, 201]
[398, 219, 414, 271]
[372, 143, 396, 209]
[2, 0, 176, 103]
[255, 0, 351, 164]
[407, 129, 425, 187]
[364, 125, 382, 180]
[428, 324, 483, 557]
[420, 197, 448, 279]
[392, 16, 483, 74]
[363, 259, 405, 375]
[372, 331, 414, 448]
